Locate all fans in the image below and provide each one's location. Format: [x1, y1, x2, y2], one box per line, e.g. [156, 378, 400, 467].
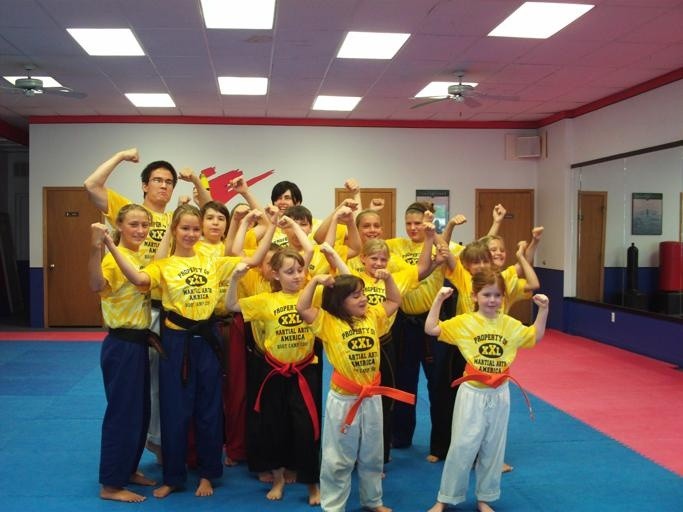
[409, 69, 523, 111]
[0, 62, 89, 102]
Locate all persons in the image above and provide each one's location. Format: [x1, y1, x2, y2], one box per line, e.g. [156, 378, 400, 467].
[421, 268, 550, 509]
[84, 145, 331, 504]
[314, 177, 545, 475]
[294, 267, 403, 507]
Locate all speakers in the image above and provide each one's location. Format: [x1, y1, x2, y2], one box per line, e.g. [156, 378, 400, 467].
[516, 136, 541, 158]
